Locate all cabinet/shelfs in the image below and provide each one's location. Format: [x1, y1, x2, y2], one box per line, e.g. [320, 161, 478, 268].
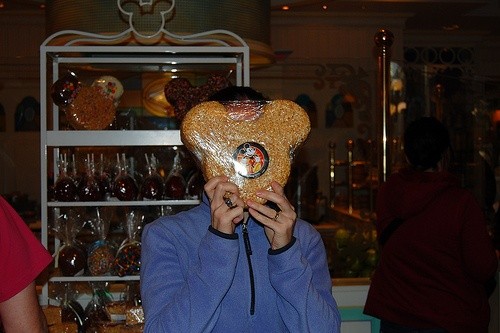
[40, 0, 250, 333]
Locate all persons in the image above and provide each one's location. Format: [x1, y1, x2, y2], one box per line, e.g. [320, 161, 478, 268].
[363, 116, 499, 333]
[0, 195, 52, 333]
[140, 87, 341, 333]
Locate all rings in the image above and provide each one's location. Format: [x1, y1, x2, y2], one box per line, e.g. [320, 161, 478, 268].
[274, 213, 279, 221]
[225, 198, 232, 208]
